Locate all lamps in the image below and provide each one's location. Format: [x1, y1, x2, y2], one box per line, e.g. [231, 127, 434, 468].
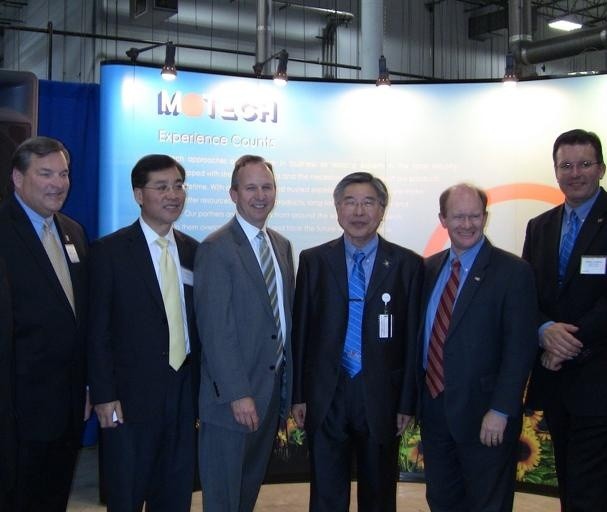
[375, 53, 393, 88]
[548, 11, 585, 34]
[498, 50, 521, 91]
[127, 37, 178, 79]
[248, 46, 290, 88]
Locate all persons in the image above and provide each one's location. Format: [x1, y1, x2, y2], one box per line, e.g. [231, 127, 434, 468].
[521, 128, 607, 512]
[0, 133, 90, 512]
[412, 182, 539, 512]
[191, 153, 298, 512]
[289, 170, 427, 512]
[90, 153, 201, 512]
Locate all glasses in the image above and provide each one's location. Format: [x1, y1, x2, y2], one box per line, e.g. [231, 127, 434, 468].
[555, 160, 599, 172]
[140, 183, 189, 195]
[339, 199, 380, 209]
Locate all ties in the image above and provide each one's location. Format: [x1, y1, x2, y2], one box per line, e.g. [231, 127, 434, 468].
[340, 248, 366, 379]
[155, 237, 187, 372]
[256, 231, 284, 375]
[557, 210, 581, 289]
[423, 258, 461, 399]
[42, 215, 76, 318]
